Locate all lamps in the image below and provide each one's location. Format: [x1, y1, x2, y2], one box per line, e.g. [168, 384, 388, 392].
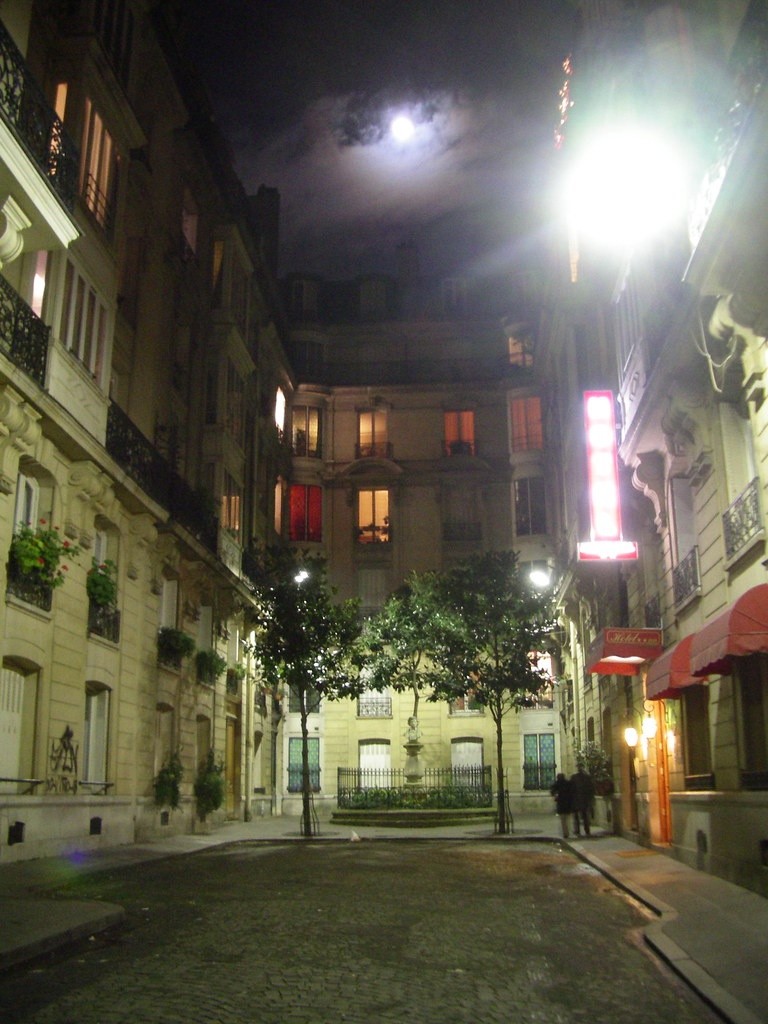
[619, 706, 643, 747]
[643, 697, 668, 739]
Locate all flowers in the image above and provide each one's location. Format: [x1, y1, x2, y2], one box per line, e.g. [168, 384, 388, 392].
[85, 553, 119, 611]
[8, 517, 80, 590]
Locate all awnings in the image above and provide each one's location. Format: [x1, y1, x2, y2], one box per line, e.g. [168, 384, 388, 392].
[586, 628, 662, 676]
[645, 582, 768, 701]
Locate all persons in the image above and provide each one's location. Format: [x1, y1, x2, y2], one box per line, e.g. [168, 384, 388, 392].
[550, 764, 594, 838]
